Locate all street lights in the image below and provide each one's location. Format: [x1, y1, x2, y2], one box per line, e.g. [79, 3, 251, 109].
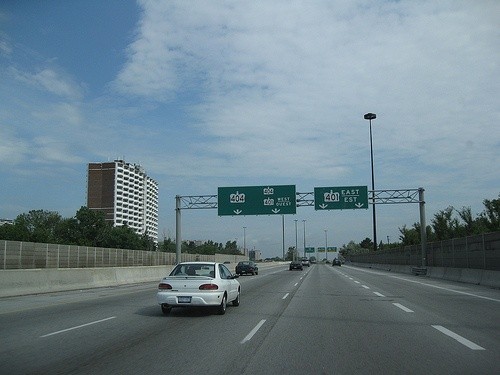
[301, 220, 307, 257]
[364, 112, 378, 251]
[294, 219, 299, 260]
[324, 230, 328, 259]
[243, 226, 247, 256]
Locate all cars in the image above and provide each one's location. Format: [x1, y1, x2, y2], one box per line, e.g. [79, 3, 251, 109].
[289, 260, 304, 271]
[332, 258, 342, 267]
[301, 256, 317, 267]
[157, 261, 242, 315]
[235, 260, 259, 276]
[323, 258, 332, 264]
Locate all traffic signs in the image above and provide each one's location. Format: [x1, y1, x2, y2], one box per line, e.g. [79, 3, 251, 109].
[313, 185, 369, 211]
[217, 184, 296, 217]
